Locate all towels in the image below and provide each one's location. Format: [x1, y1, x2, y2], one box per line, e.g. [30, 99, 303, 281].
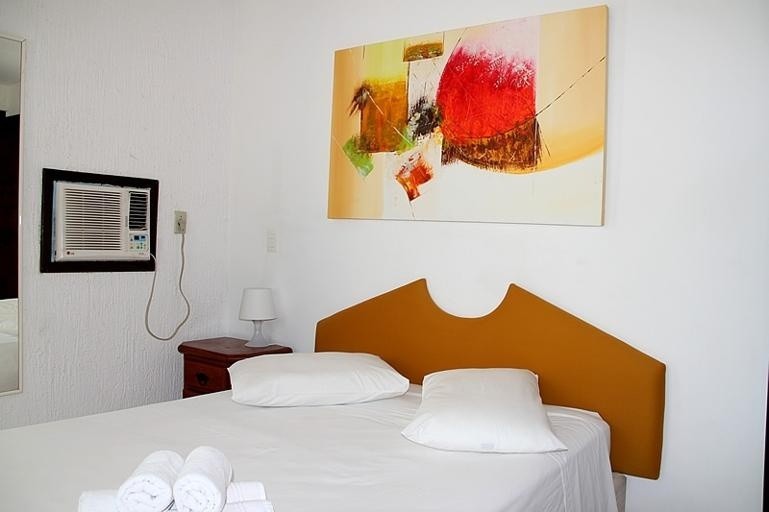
[77, 444, 275, 511]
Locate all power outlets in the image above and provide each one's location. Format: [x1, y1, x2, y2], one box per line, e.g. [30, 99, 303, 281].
[174, 210, 187, 235]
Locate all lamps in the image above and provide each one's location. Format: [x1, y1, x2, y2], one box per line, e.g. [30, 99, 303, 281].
[238, 287, 279, 348]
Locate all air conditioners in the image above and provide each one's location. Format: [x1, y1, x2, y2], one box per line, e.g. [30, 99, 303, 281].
[50, 179, 152, 263]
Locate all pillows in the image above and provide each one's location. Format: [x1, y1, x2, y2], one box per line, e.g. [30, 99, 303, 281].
[399, 365, 568, 454]
[228, 351, 412, 408]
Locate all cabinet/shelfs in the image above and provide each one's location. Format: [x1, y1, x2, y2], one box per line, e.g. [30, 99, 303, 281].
[177, 335, 293, 399]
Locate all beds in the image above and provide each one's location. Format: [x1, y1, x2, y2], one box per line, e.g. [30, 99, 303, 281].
[0, 278, 665, 511]
